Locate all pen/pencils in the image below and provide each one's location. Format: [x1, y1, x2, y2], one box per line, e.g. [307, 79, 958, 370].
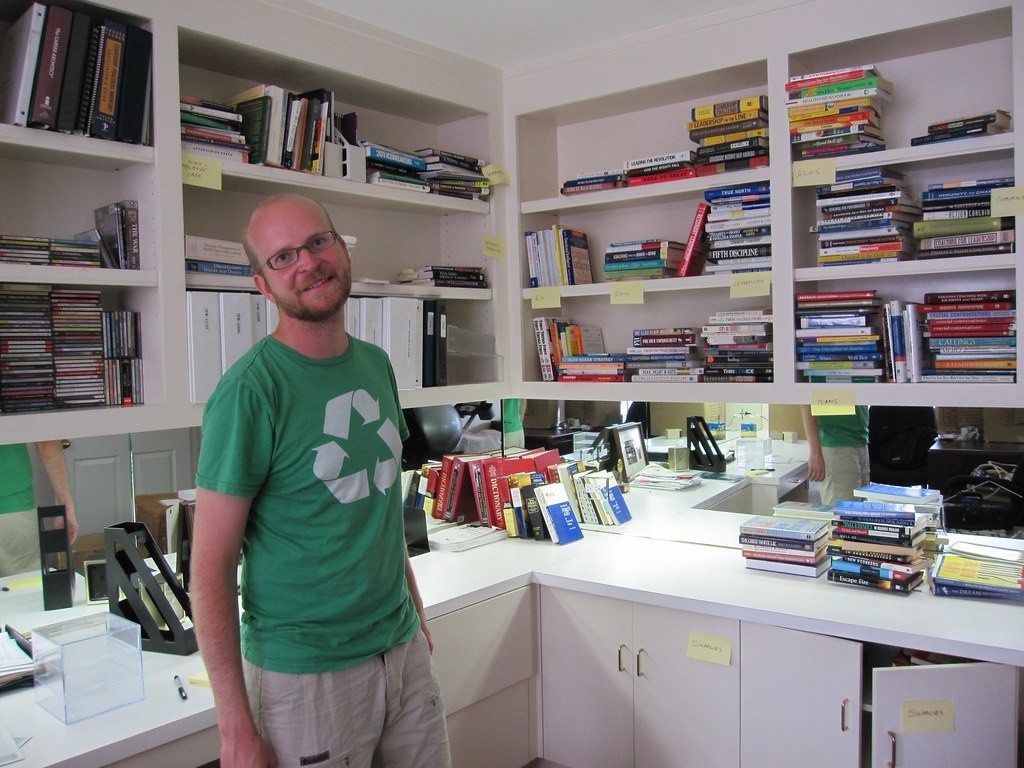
[174, 675, 187, 700]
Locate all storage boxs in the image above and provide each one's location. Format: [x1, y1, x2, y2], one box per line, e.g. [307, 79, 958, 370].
[69, 531, 129, 578]
[737, 437, 774, 471]
[32, 612, 145, 725]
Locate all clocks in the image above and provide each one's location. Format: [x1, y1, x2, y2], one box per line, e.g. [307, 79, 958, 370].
[668, 448, 690, 472]
[141, 572, 186, 631]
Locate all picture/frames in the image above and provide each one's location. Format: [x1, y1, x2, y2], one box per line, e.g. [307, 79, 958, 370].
[599, 425, 617, 472]
[613, 422, 649, 483]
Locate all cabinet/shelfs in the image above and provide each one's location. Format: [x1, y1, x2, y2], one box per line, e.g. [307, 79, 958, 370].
[532, 584, 1024, 768]
[927, 438, 1024, 496]
[135, 490, 179, 558]
[582, 468, 773, 550]
[103, 584, 544, 768]
[503, 0, 1024, 409]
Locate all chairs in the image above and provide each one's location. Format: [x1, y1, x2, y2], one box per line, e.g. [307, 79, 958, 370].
[944, 454, 1024, 504]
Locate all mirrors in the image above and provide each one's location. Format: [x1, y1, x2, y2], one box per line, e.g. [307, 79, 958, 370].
[0, 397, 504, 604]
[501, 398, 1024, 541]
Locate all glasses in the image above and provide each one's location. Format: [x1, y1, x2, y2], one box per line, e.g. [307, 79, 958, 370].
[256, 229, 339, 275]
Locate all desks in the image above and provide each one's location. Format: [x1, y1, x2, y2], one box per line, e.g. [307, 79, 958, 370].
[524, 426, 601, 456]
[0, 0, 510, 447]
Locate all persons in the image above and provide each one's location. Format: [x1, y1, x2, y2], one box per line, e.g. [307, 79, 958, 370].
[503, 399, 528, 449]
[799, 405, 872, 505]
[0, 440, 79, 577]
[188, 195, 451, 768]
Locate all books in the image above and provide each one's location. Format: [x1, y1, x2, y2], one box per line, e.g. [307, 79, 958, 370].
[395, 265, 488, 287]
[0, 281, 144, 415]
[179, 83, 490, 202]
[533, 308, 773, 381]
[482, 445, 633, 526]
[738, 516, 833, 578]
[182, 291, 447, 404]
[782, 64, 1011, 161]
[557, 94, 770, 198]
[795, 289, 1016, 382]
[184, 235, 255, 277]
[815, 168, 1015, 264]
[470, 456, 584, 546]
[825, 499, 934, 593]
[523, 185, 772, 288]
[401, 450, 490, 523]
[852, 484, 950, 561]
[931, 555, 1024, 600]
[773, 501, 843, 556]
[0, 200, 142, 270]
[0, 0, 153, 145]
